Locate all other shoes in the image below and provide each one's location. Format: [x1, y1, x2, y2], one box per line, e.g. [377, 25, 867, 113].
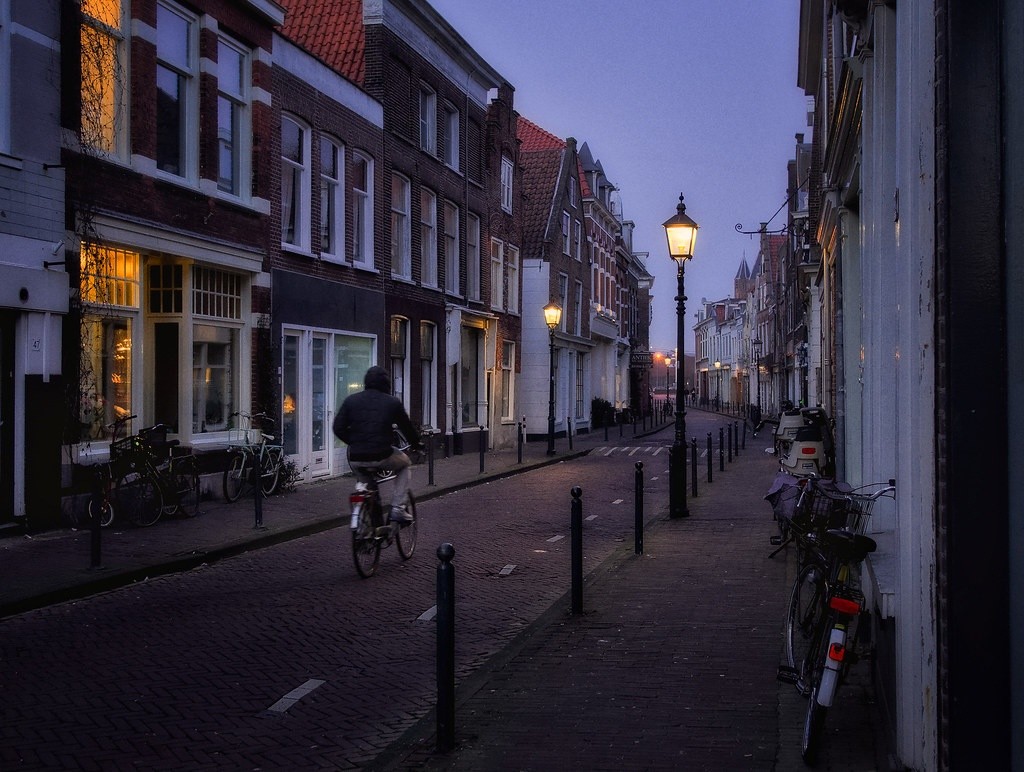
[387, 507, 414, 522]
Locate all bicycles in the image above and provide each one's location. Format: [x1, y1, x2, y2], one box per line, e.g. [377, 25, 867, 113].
[222, 411, 285, 503]
[110, 424, 201, 526]
[86, 414, 137, 528]
[350, 433, 426, 580]
[774, 470, 899, 769]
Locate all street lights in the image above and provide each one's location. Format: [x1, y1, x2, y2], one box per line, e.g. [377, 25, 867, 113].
[664, 353, 673, 416]
[714, 358, 721, 411]
[543, 294, 564, 456]
[661, 192, 697, 520]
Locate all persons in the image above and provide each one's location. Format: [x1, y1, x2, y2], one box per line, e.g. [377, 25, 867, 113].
[333, 366, 418, 526]
